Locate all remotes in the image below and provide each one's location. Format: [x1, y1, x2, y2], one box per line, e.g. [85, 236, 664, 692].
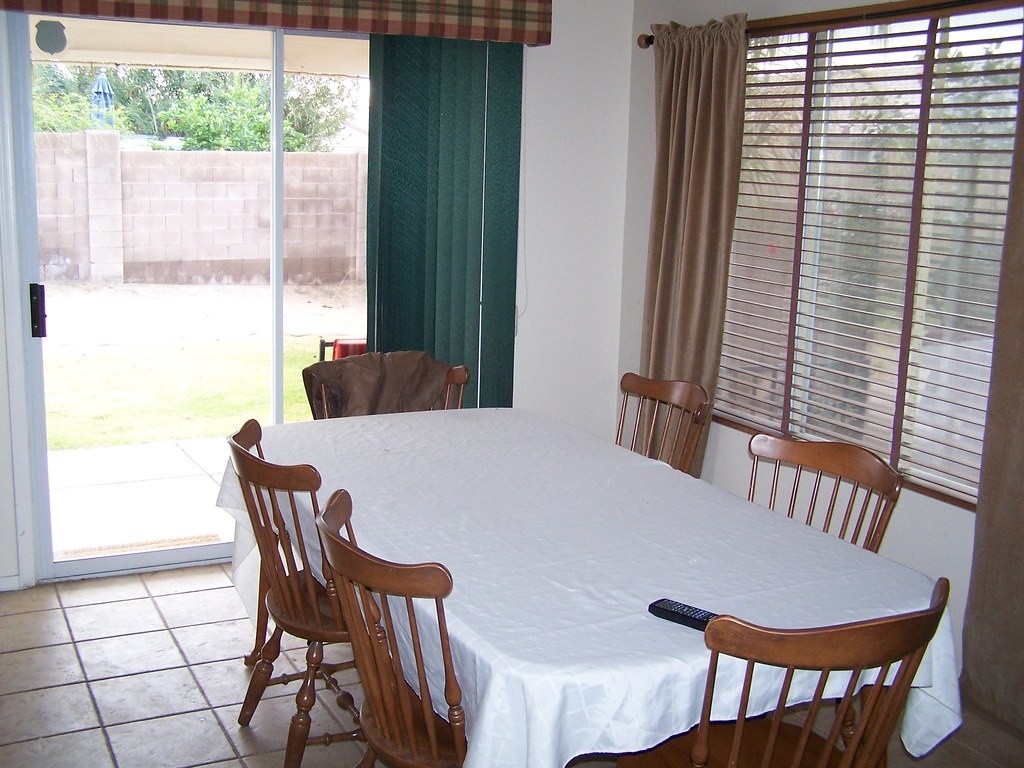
[648, 599, 719, 632]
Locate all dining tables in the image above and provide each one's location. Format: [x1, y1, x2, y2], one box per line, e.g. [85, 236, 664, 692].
[215, 406, 963, 768]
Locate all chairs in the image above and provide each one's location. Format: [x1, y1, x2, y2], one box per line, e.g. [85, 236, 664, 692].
[741, 434, 902, 557]
[225, 419, 469, 768]
[614, 577, 953, 767]
[309, 348, 469, 420]
[612, 373, 710, 473]
